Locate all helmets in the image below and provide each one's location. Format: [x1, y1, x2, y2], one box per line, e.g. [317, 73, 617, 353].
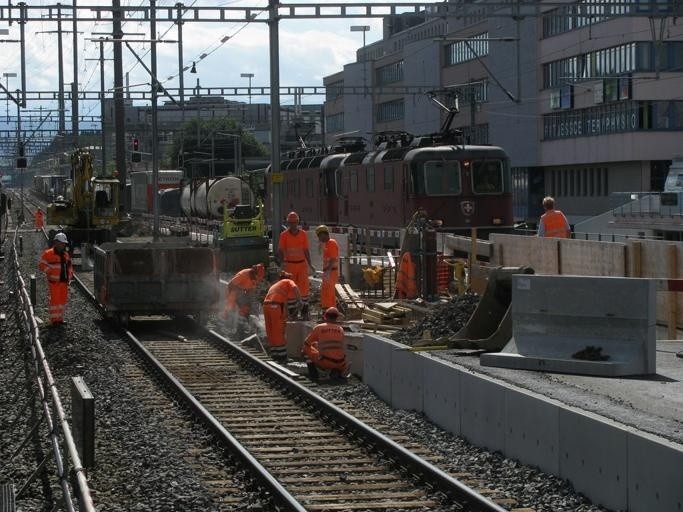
[323, 306, 341, 322]
[252, 262, 265, 280]
[314, 224, 330, 236]
[53, 233, 70, 243]
[285, 211, 300, 224]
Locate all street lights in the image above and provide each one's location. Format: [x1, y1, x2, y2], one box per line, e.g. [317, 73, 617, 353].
[241, 73, 255, 105]
[350, 25, 371, 89]
[3, 73, 17, 124]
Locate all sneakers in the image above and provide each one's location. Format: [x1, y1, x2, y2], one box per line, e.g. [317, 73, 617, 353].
[308, 362, 341, 383]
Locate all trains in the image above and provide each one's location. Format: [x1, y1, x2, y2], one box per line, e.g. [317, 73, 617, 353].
[125, 169, 271, 273]
[246, 143, 514, 272]
[31, 174, 68, 200]
[2, 176, 12, 187]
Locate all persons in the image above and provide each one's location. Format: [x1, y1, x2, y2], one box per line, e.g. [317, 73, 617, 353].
[222, 263, 264, 324]
[38, 233, 73, 329]
[303, 307, 348, 379]
[538, 196, 571, 238]
[263, 271, 303, 363]
[278, 212, 340, 323]
[34, 207, 44, 232]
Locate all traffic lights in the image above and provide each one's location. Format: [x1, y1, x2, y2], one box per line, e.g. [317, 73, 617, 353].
[132, 138, 138, 150]
[16, 142, 27, 169]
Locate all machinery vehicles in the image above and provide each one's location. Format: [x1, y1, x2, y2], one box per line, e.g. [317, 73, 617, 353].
[46, 146, 122, 242]
